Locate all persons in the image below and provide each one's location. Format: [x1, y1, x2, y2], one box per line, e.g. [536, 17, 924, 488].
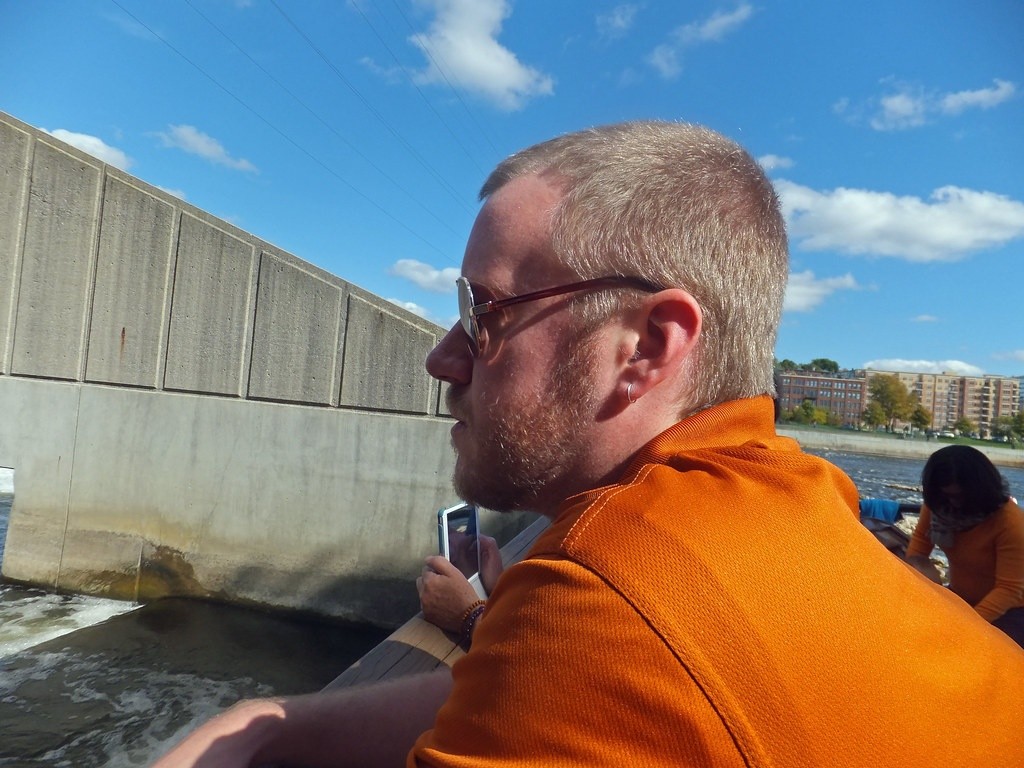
[416, 534, 505, 642]
[905, 445, 1024, 647]
[144, 121, 1024, 768]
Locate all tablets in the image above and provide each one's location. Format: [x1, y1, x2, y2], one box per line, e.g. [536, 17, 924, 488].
[438, 500, 481, 583]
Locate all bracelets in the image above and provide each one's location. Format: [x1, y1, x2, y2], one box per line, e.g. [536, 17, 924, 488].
[458, 600, 486, 645]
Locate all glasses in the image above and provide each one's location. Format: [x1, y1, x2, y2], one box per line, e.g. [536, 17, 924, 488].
[454, 275, 663, 362]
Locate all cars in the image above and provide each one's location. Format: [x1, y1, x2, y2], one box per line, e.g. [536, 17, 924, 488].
[937, 431, 954, 438]
